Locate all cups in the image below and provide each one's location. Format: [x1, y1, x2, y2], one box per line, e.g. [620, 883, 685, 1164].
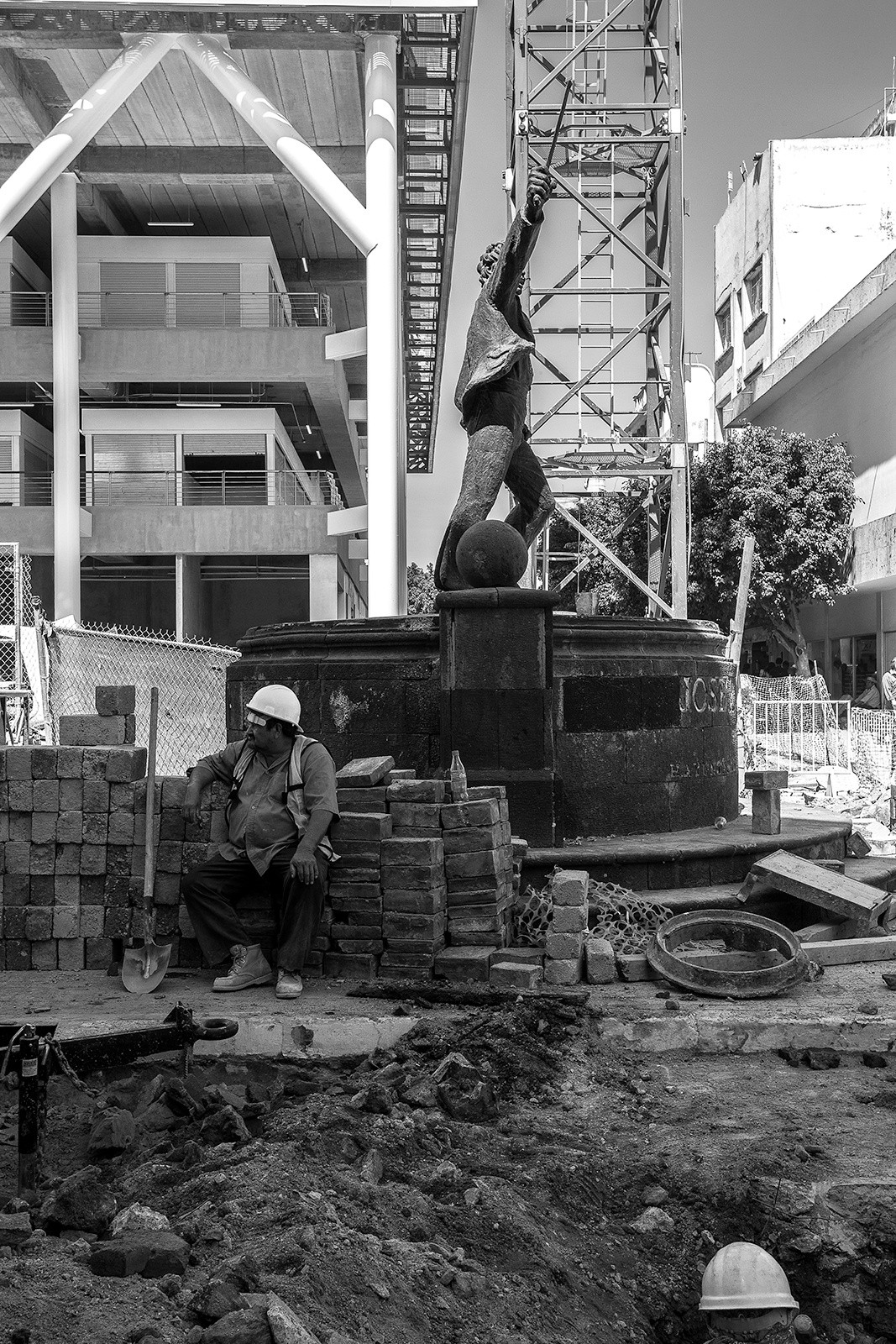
[574, 592, 592, 616]
[592, 592, 598, 616]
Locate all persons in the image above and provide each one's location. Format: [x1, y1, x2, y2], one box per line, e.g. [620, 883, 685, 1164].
[181, 684, 342, 999]
[880, 657, 896, 710]
[850, 677, 880, 710]
[831, 656, 842, 701]
[758, 658, 824, 677]
[434, 164, 557, 591]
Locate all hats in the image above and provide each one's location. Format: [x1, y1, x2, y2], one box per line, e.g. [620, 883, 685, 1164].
[864, 677, 878, 683]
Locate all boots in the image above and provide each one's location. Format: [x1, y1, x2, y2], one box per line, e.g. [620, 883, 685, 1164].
[275, 967, 303, 998]
[213, 943, 274, 991]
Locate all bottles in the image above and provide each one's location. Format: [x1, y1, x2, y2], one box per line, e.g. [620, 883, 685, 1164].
[450, 750, 470, 804]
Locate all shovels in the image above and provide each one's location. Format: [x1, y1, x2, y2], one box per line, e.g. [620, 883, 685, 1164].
[121, 687, 173, 994]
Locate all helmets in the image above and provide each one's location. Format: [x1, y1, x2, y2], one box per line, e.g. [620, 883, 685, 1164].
[246, 685, 304, 733]
